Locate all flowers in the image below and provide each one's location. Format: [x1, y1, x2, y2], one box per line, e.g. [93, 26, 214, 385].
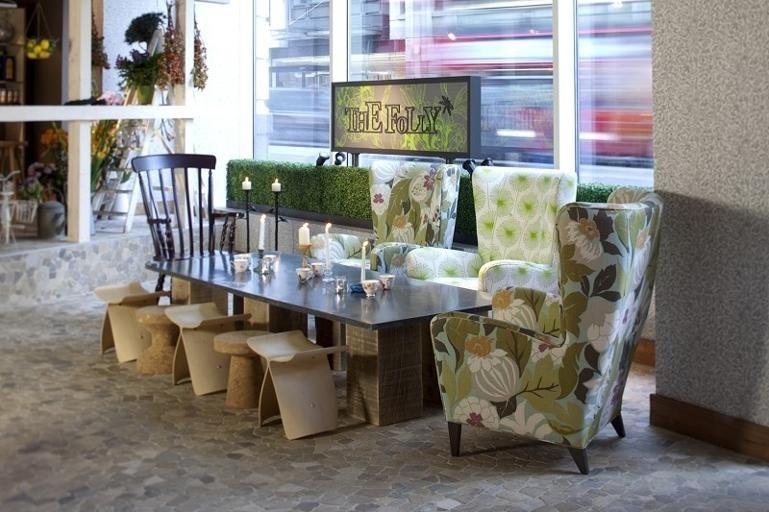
[25, 38, 52, 59]
[114, 49, 169, 96]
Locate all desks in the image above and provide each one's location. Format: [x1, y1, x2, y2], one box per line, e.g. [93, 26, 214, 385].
[145, 250, 492, 426]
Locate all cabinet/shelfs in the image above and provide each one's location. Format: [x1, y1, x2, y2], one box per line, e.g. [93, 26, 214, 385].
[0, 9, 26, 178]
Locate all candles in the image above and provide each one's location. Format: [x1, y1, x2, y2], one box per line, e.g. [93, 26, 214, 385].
[272, 179, 281, 190]
[298, 222, 310, 245]
[360, 241, 368, 282]
[323, 223, 333, 266]
[259, 214, 265, 249]
[242, 177, 252, 189]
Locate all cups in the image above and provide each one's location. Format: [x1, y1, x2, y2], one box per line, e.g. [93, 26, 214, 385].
[262, 255, 276, 274]
[335, 274, 395, 296]
[296, 262, 325, 283]
[229, 254, 251, 272]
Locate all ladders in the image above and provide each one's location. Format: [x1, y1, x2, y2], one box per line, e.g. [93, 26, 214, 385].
[92, 30, 208, 234]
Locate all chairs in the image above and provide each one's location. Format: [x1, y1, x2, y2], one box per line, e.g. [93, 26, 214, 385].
[132, 154, 243, 306]
[405, 166, 577, 297]
[430, 185, 664, 476]
[312, 160, 462, 274]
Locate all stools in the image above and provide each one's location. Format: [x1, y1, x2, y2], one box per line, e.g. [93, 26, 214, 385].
[247, 329, 349, 440]
[134, 304, 181, 374]
[214, 330, 274, 409]
[95, 281, 171, 362]
[165, 301, 252, 395]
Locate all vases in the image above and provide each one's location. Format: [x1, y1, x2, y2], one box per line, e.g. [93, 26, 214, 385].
[136, 85, 155, 104]
